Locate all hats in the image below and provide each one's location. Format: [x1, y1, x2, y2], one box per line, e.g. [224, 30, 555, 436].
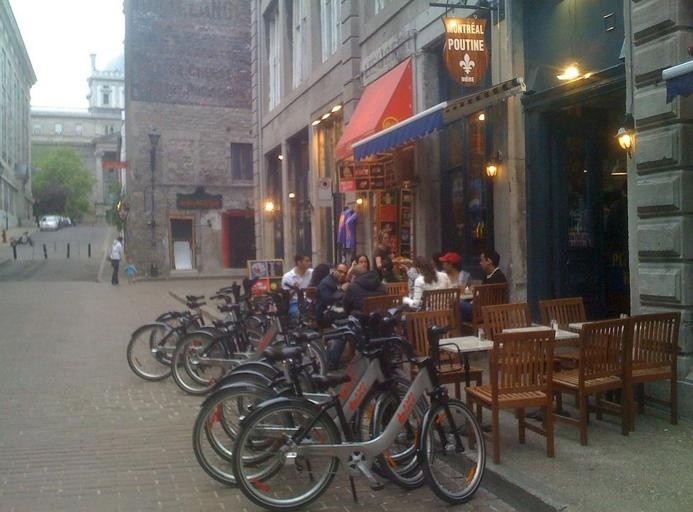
[438, 252, 462, 265]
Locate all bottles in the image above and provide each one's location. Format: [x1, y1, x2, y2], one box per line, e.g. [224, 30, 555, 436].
[551, 319, 558, 332]
[477, 327, 485, 342]
[619, 312, 629, 320]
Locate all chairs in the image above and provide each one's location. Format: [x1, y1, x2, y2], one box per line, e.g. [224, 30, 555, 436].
[295, 279, 682, 466]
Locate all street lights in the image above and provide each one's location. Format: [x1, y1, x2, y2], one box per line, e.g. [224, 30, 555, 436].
[144, 127, 162, 278]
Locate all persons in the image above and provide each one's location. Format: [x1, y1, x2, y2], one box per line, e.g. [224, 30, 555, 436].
[280, 250, 509, 377]
[123, 259, 137, 285]
[109, 236, 125, 284]
[373, 230, 396, 270]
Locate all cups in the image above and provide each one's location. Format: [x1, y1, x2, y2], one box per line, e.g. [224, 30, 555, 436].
[457, 285, 465, 297]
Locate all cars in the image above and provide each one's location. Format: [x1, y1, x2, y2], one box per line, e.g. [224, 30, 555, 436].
[38, 214, 72, 232]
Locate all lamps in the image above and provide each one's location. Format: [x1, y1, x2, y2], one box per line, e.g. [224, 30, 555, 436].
[612, 125, 636, 160]
[482, 147, 505, 177]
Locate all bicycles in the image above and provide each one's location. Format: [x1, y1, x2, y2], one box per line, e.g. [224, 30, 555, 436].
[9, 230, 33, 247]
[229, 322, 486, 510]
[126, 272, 438, 491]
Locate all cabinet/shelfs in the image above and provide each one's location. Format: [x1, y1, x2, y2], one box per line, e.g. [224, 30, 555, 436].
[374, 187, 412, 259]
